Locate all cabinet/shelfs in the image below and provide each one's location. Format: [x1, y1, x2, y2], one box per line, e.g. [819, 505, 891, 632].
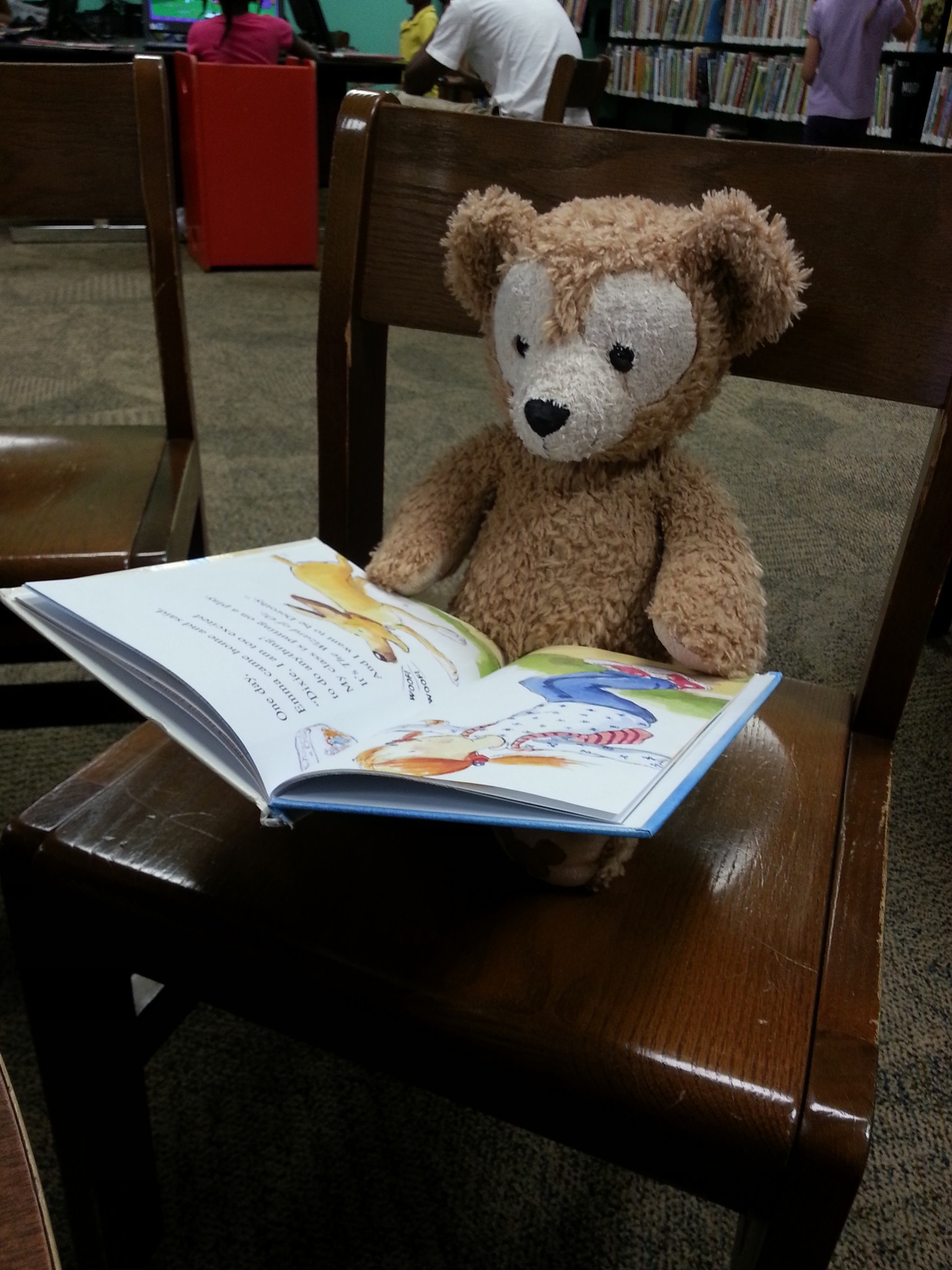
[561, 0, 952, 152]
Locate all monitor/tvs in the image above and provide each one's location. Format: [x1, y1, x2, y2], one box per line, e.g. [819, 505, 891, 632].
[141, 0, 284, 36]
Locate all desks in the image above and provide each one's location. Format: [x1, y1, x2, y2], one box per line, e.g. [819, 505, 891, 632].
[0, 29, 408, 187]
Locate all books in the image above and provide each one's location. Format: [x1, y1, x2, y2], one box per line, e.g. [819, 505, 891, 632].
[0, 536, 783, 830]
[557, 0, 815, 124]
[866, 0, 952, 150]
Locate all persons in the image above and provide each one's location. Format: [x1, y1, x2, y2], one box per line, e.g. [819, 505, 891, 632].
[404, 0, 595, 128]
[801, 0, 916, 148]
[398, 0, 440, 99]
[186, 0, 325, 69]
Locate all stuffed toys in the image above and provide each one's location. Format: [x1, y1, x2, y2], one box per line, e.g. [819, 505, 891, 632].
[369, 182, 815, 890]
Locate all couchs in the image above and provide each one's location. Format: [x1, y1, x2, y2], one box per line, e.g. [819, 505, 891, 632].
[175, 51, 320, 272]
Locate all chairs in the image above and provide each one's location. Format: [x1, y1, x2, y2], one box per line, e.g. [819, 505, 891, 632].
[0, 90, 952, 1270]
[0, 53, 211, 723]
[540, 54, 614, 124]
